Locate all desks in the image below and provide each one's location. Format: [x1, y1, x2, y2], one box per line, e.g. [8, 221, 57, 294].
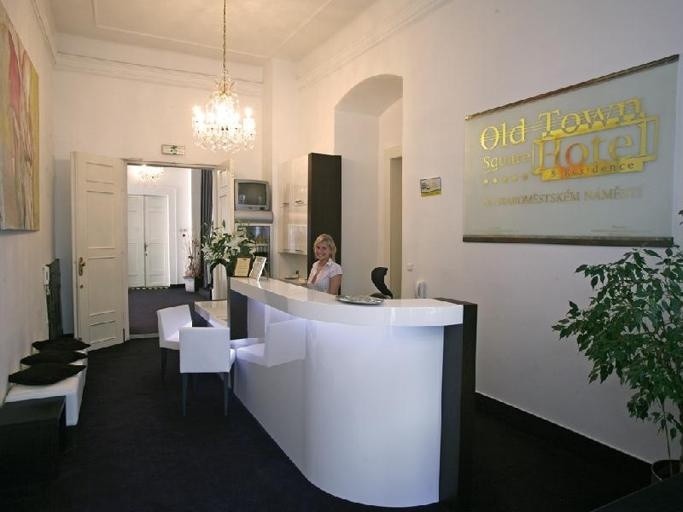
[0, 396, 67, 510]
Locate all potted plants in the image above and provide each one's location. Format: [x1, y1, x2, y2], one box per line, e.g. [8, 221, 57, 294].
[548, 210, 682, 483]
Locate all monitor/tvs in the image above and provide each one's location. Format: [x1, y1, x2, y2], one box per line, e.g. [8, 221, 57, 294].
[234, 178, 270, 211]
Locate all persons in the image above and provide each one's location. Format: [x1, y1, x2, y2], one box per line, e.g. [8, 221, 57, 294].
[305, 234, 344, 296]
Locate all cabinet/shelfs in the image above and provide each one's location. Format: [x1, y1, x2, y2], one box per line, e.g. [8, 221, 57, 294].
[276, 156, 308, 255]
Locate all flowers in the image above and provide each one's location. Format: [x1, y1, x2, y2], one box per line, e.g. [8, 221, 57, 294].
[186, 222, 255, 278]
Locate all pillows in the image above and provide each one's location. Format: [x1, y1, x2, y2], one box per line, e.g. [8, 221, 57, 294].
[8, 333, 88, 386]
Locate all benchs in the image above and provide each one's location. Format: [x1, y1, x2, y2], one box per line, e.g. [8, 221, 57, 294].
[1, 344, 88, 428]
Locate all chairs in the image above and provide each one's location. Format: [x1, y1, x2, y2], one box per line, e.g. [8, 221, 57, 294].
[154, 302, 237, 418]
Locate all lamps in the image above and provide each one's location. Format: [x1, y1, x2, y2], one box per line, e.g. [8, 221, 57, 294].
[192, 5, 257, 154]
